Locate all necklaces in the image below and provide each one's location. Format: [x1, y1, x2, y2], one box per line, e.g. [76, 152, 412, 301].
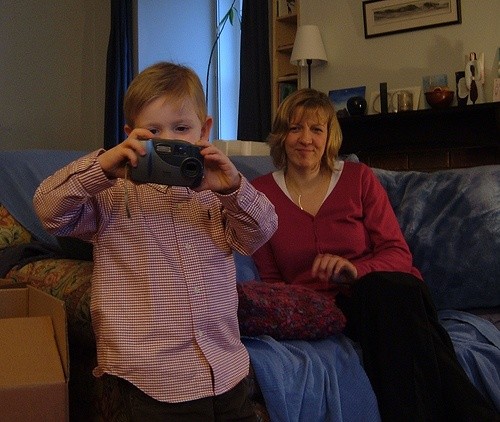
[288, 177, 303, 209]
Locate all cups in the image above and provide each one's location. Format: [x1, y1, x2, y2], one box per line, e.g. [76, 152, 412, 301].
[398, 93, 413, 112]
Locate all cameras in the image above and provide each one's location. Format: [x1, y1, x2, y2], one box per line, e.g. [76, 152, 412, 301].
[124, 137, 206, 190]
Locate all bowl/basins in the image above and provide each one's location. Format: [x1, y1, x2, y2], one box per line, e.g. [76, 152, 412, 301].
[425, 88, 454, 108]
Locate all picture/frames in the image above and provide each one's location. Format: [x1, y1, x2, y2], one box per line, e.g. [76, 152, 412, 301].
[362, 0, 462, 39]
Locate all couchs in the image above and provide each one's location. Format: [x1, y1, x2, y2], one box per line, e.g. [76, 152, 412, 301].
[0, 149, 500, 422]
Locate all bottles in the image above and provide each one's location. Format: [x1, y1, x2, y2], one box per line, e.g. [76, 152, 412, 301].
[347, 96, 366, 115]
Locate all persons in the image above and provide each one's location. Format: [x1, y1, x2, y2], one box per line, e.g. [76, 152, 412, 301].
[33, 61, 279, 422]
[220, 88, 500, 422]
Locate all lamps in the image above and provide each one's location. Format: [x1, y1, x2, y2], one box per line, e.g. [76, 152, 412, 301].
[288, 24, 328, 89]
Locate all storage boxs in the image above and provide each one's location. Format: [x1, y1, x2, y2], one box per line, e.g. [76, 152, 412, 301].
[0, 283, 70, 422]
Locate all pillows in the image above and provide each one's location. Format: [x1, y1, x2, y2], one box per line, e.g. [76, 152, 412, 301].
[235, 277, 348, 343]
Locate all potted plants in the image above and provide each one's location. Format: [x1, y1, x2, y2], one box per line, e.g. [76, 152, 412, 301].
[205, 0, 271, 157]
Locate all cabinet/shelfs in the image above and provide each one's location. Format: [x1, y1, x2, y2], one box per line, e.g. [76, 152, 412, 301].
[275, 0, 300, 109]
[336, 100, 500, 172]
